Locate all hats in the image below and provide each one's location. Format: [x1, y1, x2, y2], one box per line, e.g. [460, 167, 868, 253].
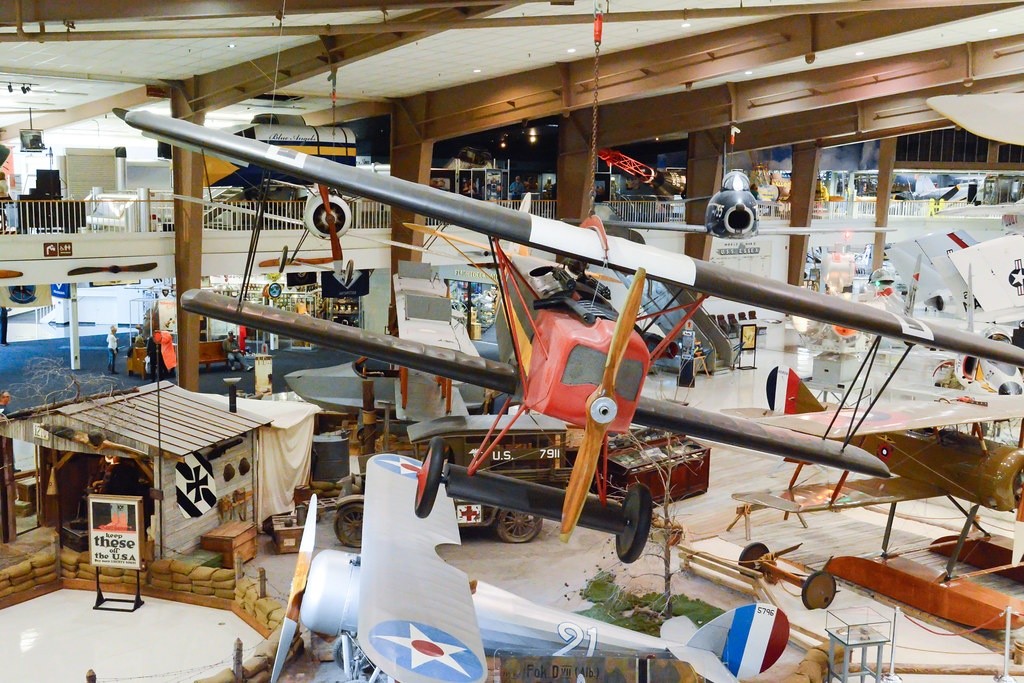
[228, 331, 233, 335]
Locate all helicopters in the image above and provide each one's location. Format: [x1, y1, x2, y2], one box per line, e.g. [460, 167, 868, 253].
[716, 364, 1024, 588]
[168, 171, 459, 262]
[590, 169, 898, 240]
[596, 147, 688, 200]
[113, 106, 1024, 560]
[265, 451, 790, 682]
[871, 249, 1024, 405]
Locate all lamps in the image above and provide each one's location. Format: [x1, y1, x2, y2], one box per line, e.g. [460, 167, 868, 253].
[21, 86, 27, 94]
[8, 85, 13, 92]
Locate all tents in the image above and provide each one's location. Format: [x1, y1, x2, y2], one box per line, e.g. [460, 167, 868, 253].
[198, 391, 322, 536]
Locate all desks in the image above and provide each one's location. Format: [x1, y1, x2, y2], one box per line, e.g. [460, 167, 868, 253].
[693, 356, 712, 379]
[828, 634, 886, 683]
[801, 378, 873, 409]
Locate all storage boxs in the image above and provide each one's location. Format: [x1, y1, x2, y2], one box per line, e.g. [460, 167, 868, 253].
[14, 500, 34, 517]
[177, 548, 224, 568]
[824, 605, 891, 647]
[199, 521, 258, 569]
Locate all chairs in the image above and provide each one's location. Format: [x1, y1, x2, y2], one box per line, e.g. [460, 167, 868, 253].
[717, 315, 731, 335]
[748, 310, 757, 319]
[728, 314, 738, 333]
[738, 312, 747, 320]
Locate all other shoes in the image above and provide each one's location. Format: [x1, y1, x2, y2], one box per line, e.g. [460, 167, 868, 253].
[232, 366, 235, 370]
[247, 366, 254, 371]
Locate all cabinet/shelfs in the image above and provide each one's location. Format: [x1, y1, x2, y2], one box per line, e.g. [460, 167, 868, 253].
[332, 297, 359, 316]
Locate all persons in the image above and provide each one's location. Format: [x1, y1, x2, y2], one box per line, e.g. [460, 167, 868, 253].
[0, 390, 12, 416]
[146, 336, 164, 384]
[509, 174, 525, 211]
[543, 177, 553, 200]
[106, 325, 119, 374]
[611, 177, 620, 201]
[222, 331, 254, 371]
[527, 176, 539, 193]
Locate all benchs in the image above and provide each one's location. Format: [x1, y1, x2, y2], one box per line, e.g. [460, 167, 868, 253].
[173, 341, 244, 372]
[127, 347, 148, 381]
